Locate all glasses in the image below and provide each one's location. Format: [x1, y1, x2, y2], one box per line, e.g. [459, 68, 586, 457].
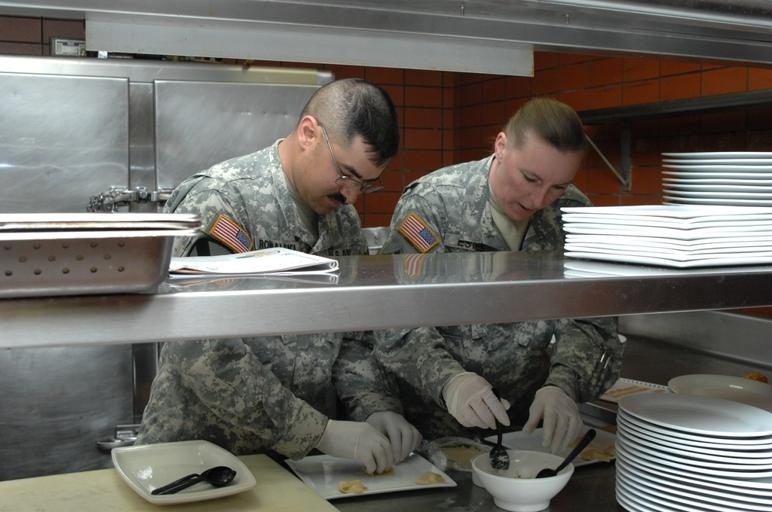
[318, 121, 386, 195]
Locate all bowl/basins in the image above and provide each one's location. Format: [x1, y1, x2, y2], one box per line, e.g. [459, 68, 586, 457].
[472, 450, 575, 510]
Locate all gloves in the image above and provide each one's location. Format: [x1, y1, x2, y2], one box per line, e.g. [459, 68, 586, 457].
[364, 411, 425, 465]
[522, 385, 584, 457]
[439, 370, 512, 430]
[314, 418, 394, 476]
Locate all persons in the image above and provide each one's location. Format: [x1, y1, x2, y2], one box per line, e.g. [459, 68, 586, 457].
[370, 97, 624, 453]
[133, 77, 424, 474]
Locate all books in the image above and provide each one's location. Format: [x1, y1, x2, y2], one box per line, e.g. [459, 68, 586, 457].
[170, 247, 340, 279]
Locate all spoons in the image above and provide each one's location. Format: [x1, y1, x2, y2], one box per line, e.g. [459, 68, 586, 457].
[536, 430, 598, 479]
[150, 464, 238, 498]
[490, 386, 513, 471]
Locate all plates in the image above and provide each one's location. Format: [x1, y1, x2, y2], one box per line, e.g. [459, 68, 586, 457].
[613, 373, 771, 510]
[564, 260, 772, 280]
[556, 151, 771, 267]
[484, 420, 616, 468]
[111, 441, 259, 507]
[284, 443, 459, 500]
